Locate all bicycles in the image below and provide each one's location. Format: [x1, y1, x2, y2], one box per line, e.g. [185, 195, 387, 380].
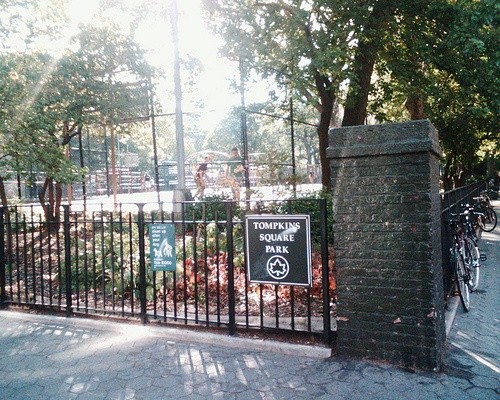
[448, 192, 497, 312]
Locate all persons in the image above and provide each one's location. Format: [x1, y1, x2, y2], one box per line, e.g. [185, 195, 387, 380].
[225, 147, 246, 205]
[191, 156, 210, 201]
[144, 172, 154, 193]
